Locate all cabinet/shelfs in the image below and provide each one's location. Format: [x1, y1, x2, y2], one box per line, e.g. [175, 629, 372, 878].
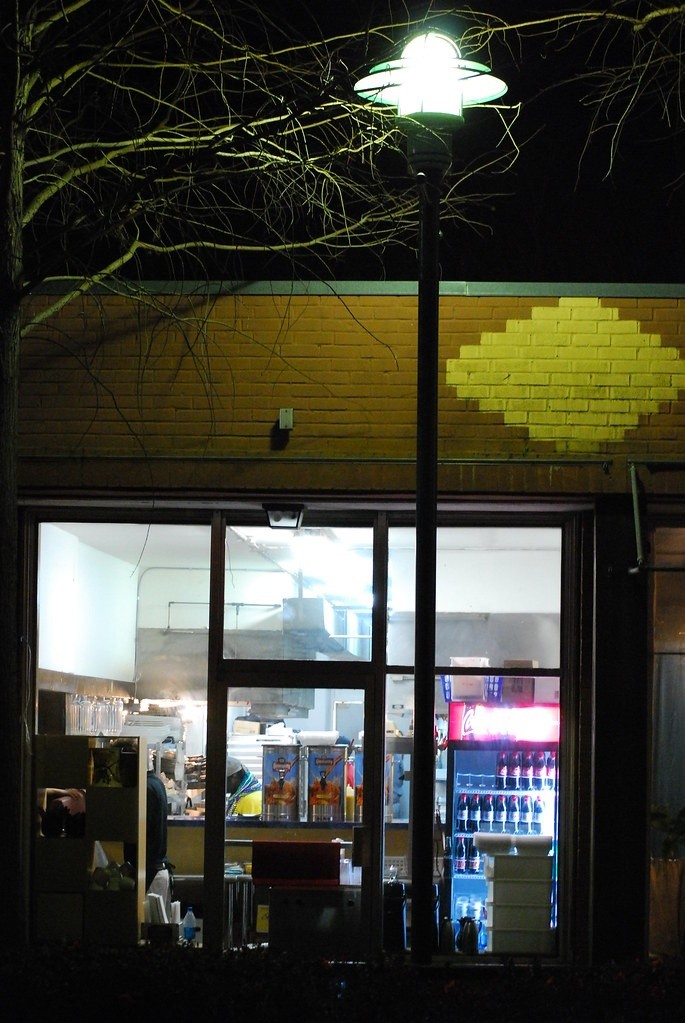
[33, 735, 147, 946]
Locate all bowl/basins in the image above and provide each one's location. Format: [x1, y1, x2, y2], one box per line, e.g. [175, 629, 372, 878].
[512, 834, 553, 855]
[473, 831, 511, 855]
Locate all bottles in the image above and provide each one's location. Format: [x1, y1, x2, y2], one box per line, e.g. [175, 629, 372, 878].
[346, 783, 354, 821]
[457, 837, 479, 875]
[245, 863, 252, 874]
[69, 694, 123, 736]
[183, 907, 197, 948]
[496, 751, 555, 790]
[384, 881, 406, 953]
[431, 884, 439, 949]
[454, 793, 543, 834]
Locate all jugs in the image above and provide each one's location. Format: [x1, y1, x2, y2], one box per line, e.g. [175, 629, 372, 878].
[442, 918, 455, 955]
[456, 917, 482, 955]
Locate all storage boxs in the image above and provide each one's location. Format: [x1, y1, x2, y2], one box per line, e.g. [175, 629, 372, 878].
[502, 659, 538, 700]
[489, 878, 554, 905]
[482, 923, 557, 955]
[482, 901, 556, 928]
[485, 853, 556, 881]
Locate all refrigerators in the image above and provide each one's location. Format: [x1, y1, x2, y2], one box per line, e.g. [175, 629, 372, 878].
[442, 702, 559, 955]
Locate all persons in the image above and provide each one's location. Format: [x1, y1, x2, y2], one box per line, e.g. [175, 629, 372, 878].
[38, 788, 81, 835]
[186, 756, 262, 814]
[109, 738, 167, 888]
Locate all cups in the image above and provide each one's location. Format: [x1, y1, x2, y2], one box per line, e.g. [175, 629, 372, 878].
[339, 858, 351, 885]
[94, 860, 135, 889]
[456, 894, 482, 919]
